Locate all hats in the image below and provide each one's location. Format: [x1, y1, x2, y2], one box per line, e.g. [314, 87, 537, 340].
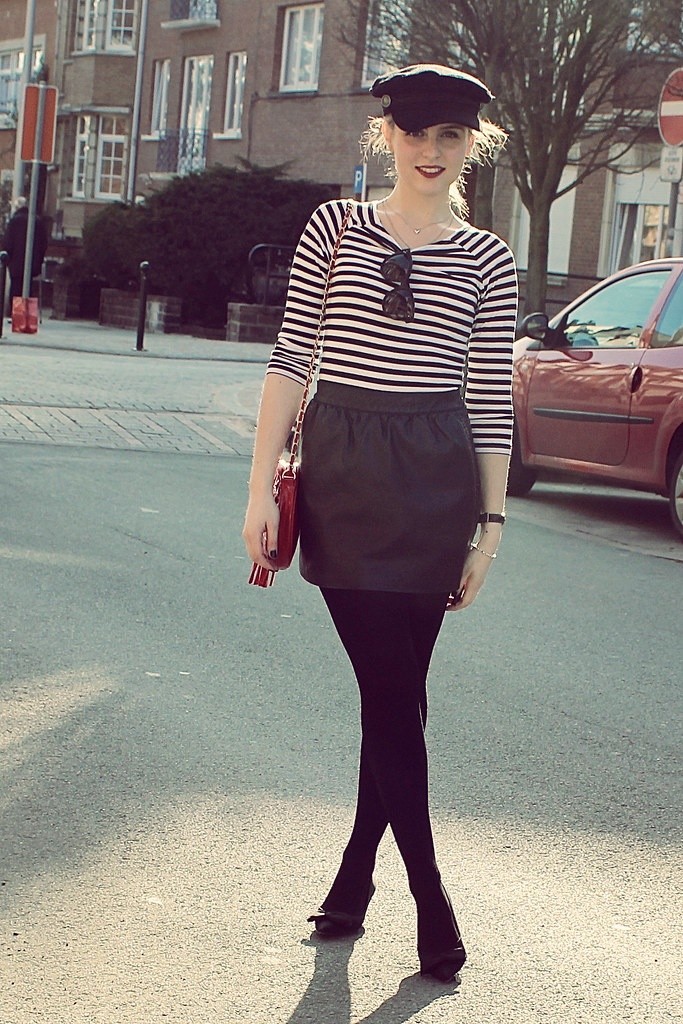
[370, 62, 494, 131]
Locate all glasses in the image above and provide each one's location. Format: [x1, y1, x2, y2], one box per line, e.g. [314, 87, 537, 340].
[379, 247, 416, 324]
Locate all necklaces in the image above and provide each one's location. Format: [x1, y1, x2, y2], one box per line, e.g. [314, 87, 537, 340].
[382, 197, 454, 251]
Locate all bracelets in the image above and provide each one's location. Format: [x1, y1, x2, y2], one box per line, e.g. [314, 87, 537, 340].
[469, 542, 497, 559]
[478, 513, 506, 526]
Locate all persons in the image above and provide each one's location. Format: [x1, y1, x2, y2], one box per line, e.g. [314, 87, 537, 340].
[4, 197, 47, 324]
[241, 62, 521, 983]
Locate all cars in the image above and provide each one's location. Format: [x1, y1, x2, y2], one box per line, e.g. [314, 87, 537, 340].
[505, 257, 683, 540]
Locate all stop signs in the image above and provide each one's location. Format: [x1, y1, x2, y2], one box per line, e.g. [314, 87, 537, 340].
[656, 67, 683, 147]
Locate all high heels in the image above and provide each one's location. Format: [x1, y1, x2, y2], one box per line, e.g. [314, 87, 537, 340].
[414, 882, 468, 980]
[305, 856, 376, 939]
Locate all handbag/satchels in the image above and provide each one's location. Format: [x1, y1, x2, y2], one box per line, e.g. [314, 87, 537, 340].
[249, 459, 299, 589]
